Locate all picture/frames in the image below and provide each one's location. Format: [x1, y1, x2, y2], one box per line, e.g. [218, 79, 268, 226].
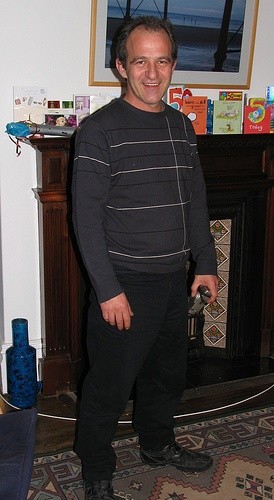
[88, 0, 260, 90]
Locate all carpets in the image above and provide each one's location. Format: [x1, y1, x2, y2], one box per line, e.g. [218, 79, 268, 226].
[28, 403, 274, 500]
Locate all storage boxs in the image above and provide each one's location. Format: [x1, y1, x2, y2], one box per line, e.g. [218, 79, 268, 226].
[182, 96, 271, 135]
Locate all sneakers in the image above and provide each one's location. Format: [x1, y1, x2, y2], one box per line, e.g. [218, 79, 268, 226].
[140, 441, 213, 473]
[84, 478, 115, 500]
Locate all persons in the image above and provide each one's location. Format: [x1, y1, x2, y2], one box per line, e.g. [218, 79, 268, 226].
[71, 16, 220, 500]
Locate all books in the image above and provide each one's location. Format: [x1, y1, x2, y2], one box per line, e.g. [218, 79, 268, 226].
[182, 89, 274, 134]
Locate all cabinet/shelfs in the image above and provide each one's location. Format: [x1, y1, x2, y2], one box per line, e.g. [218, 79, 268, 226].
[17, 133, 274, 414]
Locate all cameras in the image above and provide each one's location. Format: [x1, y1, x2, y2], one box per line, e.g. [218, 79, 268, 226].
[187, 286, 211, 317]
